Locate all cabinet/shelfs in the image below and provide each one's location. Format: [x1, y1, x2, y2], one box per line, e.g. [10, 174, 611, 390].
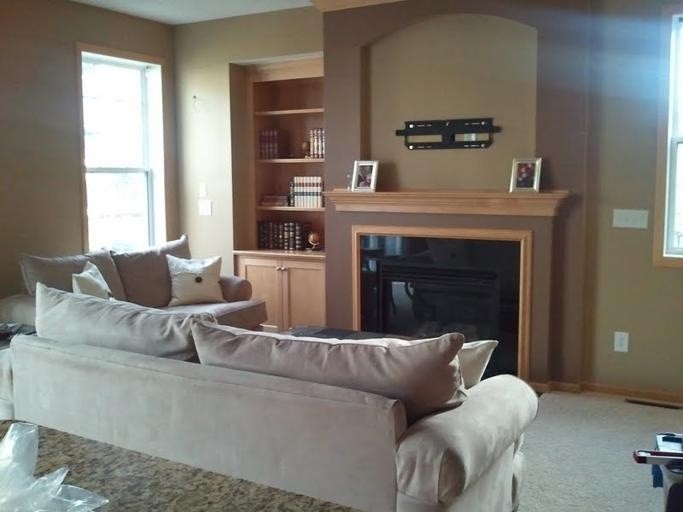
[233, 58, 325, 336]
[323, 189, 569, 394]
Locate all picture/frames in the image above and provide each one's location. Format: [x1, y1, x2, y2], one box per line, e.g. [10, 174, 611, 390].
[510, 156, 543, 191]
[351, 159, 379, 191]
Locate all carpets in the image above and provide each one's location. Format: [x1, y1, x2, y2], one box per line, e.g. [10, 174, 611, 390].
[521, 388, 681, 512]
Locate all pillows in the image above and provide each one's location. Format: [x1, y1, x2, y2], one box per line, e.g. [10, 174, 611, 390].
[191, 317, 467, 426]
[110, 233, 191, 307]
[165, 253, 228, 307]
[72, 260, 112, 300]
[36, 281, 216, 362]
[17, 250, 127, 301]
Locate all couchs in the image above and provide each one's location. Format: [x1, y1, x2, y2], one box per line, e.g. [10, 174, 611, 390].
[10, 283, 542, 512]
[1, 274, 269, 342]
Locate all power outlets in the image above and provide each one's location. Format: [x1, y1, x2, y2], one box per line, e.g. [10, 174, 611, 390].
[613, 331, 629, 353]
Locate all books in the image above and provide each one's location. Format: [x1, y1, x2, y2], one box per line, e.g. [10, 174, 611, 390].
[289, 176, 323, 206]
[309, 128, 325, 159]
[259, 128, 288, 159]
[258, 220, 312, 250]
[259, 195, 289, 208]
